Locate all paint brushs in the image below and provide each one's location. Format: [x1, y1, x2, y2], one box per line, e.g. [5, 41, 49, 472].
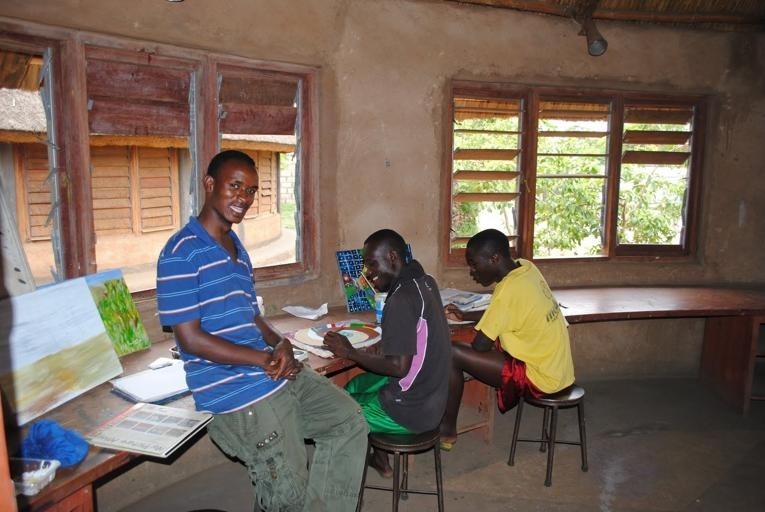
[359, 270, 376, 294]
[333, 327, 344, 333]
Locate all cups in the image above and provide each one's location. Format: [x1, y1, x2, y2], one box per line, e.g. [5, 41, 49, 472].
[375, 293, 387, 324]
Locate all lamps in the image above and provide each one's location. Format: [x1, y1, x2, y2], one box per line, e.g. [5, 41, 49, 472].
[576, 10, 608, 57]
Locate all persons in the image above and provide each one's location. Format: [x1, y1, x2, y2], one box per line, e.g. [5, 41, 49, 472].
[322, 229, 453, 477]
[156, 149, 372, 512]
[437, 228, 575, 451]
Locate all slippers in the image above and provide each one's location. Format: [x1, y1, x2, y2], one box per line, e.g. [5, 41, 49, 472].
[368, 453, 393, 480]
[439, 442, 453, 451]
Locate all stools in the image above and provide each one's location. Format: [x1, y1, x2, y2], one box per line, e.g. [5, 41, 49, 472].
[355, 431, 446, 512]
[508, 385, 589, 487]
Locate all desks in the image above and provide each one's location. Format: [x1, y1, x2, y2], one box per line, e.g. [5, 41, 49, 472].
[7, 283, 765, 512]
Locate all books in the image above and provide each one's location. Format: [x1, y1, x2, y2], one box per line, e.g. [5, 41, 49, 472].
[473, 292, 492, 308]
[83, 401, 214, 459]
[449, 293, 482, 311]
